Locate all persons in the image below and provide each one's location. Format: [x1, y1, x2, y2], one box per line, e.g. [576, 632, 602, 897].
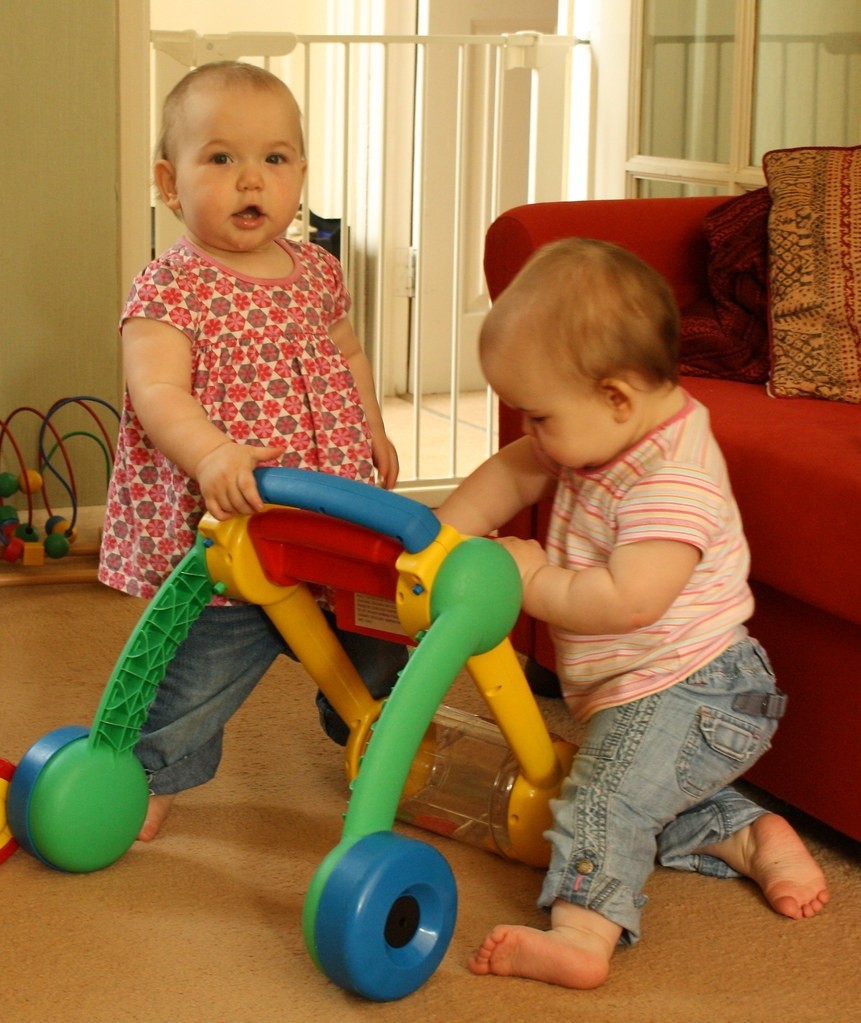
[432, 236, 831, 990]
[99, 61, 399, 845]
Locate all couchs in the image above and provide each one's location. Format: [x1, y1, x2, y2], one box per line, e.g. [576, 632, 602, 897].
[479, 196, 861, 837]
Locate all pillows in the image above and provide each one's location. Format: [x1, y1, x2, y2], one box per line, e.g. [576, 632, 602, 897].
[760, 142, 861, 402]
